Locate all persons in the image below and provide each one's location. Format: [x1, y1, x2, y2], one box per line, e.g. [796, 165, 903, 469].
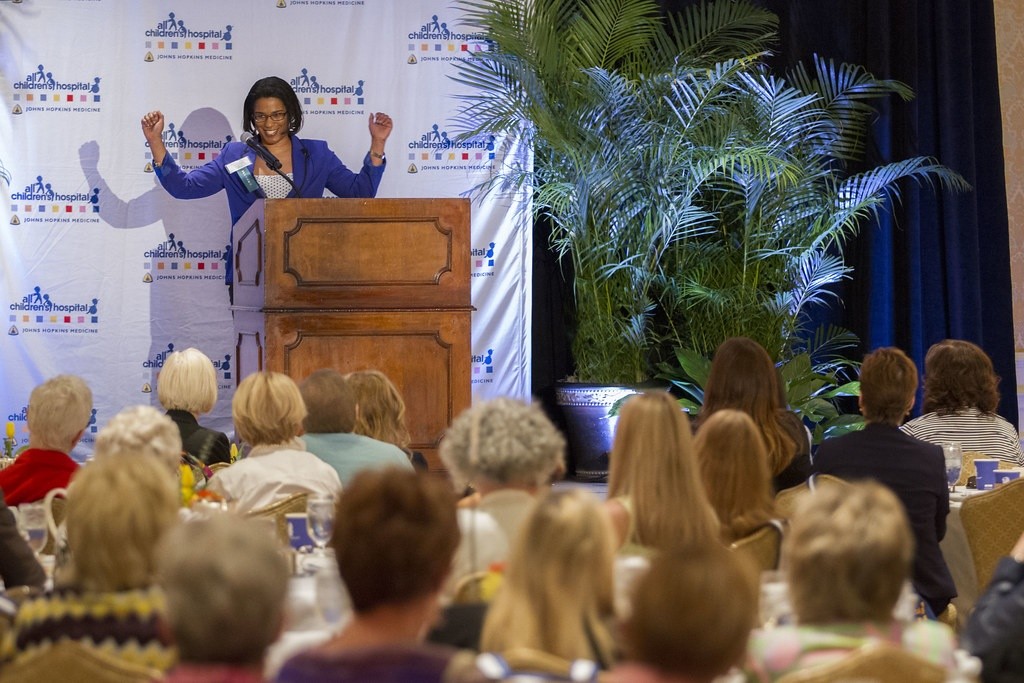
[140, 76, 394, 319]
[0, 336, 1024, 683]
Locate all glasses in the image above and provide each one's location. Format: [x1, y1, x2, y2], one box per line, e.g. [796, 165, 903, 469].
[251, 111, 287, 123]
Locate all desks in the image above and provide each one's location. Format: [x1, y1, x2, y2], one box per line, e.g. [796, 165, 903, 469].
[938, 486, 990, 630]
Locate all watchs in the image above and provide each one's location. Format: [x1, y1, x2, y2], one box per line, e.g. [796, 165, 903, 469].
[371, 150, 385, 159]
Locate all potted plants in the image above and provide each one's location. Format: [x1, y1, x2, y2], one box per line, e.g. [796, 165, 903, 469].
[432, 0, 805, 470]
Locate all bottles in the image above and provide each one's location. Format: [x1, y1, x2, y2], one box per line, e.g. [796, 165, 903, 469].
[19, 504, 48, 556]
[941, 441, 962, 493]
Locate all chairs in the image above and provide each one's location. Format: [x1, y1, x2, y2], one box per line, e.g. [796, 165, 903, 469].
[1, 451, 1024, 683]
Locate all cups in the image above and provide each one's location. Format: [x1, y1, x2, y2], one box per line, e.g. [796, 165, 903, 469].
[285, 513, 314, 554]
[305, 499, 336, 549]
[945, 459, 961, 485]
[316, 577, 344, 623]
[972, 458, 1020, 490]
[1012, 467, 1024, 477]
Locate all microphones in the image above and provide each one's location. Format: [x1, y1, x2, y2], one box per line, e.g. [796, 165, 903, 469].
[240, 132, 282, 169]
[292, 148, 308, 198]
